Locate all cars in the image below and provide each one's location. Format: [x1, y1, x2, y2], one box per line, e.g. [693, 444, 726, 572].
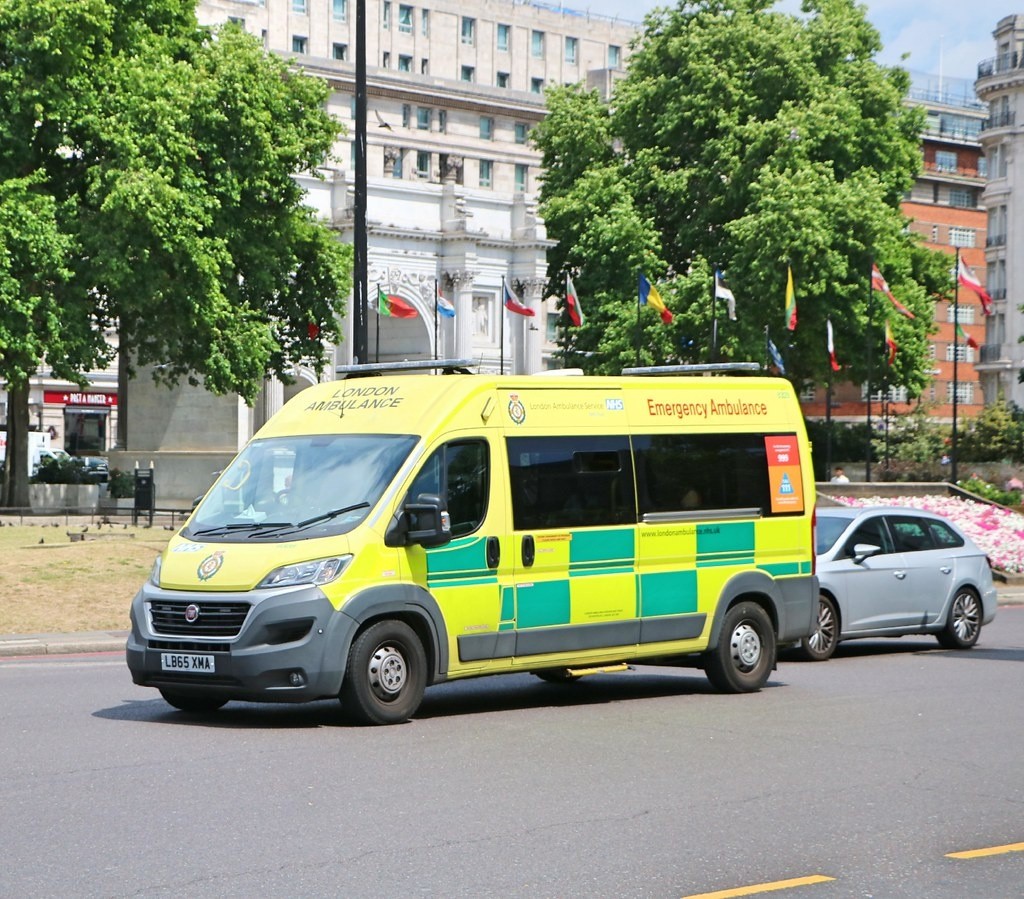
[31, 455, 109, 485]
[780, 504, 998, 660]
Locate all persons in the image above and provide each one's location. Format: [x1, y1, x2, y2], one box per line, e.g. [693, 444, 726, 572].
[830, 467, 850, 484]
[334, 456, 408, 536]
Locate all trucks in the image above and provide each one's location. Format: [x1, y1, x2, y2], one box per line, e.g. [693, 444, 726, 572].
[0, 427, 70, 480]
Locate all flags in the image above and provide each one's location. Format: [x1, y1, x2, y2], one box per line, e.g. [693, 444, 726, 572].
[713, 268, 737, 321]
[565, 273, 585, 328]
[308, 320, 318, 339]
[827, 319, 841, 372]
[432, 283, 456, 318]
[637, 273, 674, 324]
[376, 289, 418, 320]
[957, 255, 994, 316]
[884, 319, 895, 367]
[956, 322, 980, 350]
[501, 280, 536, 318]
[871, 263, 916, 319]
[768, 340, 786, 375]
[785, 266, 797, 331]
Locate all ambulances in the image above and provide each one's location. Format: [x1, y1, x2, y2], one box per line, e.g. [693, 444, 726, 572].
[124, 362, 824, 727]
